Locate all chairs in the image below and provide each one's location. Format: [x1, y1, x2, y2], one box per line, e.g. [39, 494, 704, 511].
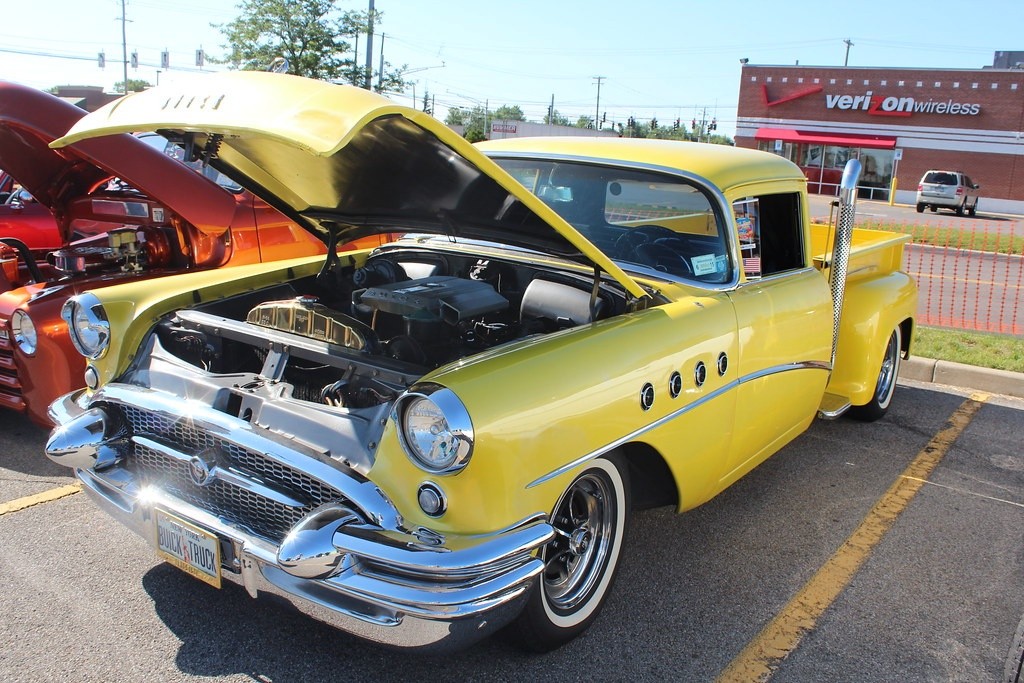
[653, 226, 722, 281]
[587, 225, 649, 264]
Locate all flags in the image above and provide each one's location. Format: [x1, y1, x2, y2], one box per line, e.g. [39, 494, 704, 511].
[743, 258, 761, 273]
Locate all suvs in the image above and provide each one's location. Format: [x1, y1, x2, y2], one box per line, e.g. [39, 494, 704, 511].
[915, 169, 980, 216]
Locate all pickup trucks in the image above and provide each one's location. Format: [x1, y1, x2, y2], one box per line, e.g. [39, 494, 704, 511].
[0, 80, 412, 433]
[40, 67, 920, 656]
